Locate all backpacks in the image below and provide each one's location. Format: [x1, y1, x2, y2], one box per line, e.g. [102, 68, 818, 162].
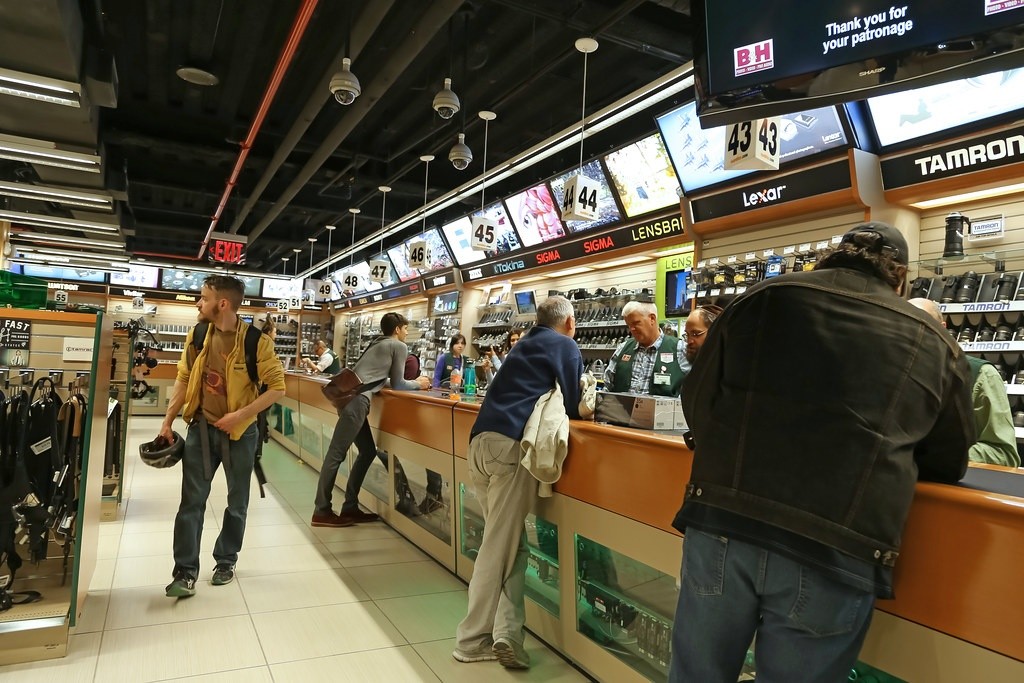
[189, 322, 273, 457]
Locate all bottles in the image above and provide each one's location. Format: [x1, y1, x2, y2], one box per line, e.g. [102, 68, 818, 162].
[464, 358, 477, 396]
[449, 365, 461, 400]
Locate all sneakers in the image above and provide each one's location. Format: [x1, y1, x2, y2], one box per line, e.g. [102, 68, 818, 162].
[452, 644, 497, 662]
[492, 637, 531, 669]
[211, 563, 236, 585]
[165, 571, 196, 596]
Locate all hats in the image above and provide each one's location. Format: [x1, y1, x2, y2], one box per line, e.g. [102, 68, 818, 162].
[701, 304, 724, 318]
[837, 221, 909, 266]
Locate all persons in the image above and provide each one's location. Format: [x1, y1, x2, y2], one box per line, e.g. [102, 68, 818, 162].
[685, 304, 723, 364]
[481, 328, 522, 386]
[603, 301, 692, 398]
[159, 275, 285, 597]
[664, 221, 974, 683]
[433, 334, 470, 388]
[375, 449, 443, 517]
[302, 340, 339, 375]
[312, 312, 431, 527]
[907, 297, 1022, 468]
[452, 296, 585, 669]
[404, 352, 420, 381]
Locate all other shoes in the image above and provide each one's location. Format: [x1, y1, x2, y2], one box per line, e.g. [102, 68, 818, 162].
[396, 500, 420, 518]
[340, 505, 379, 523]
[419, 496, 444, 513]
[311, 512, 354, 527]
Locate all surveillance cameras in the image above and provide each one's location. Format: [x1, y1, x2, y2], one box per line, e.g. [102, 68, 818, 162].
[432, 77, 461, 120]
[328, 56, 362, 104]
[448, 134, 474, 169]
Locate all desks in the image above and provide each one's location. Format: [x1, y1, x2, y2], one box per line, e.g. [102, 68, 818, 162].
[571, 290, 653, 350]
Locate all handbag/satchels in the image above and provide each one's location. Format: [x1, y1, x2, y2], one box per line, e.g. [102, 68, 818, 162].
[320, 368, 364, 408]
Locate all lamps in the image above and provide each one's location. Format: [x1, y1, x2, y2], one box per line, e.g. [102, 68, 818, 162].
[0, 133, 102, 174]
[0, 207, 121, 238]
[0, 67, 83, 110]
[0, 180, 116, 211]
[6, 230, 127, 253]
[14, 243, 131, 264]
[6, 255, 130, 273]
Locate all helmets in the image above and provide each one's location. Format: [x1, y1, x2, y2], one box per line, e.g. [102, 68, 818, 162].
[139, 431, 185, 468]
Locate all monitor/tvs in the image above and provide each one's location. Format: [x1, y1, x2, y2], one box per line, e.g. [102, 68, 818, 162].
[688, 0, 1024, 130]
[432, 291, 461, 315]
[666, 268, 693, 317]
[514, 290, 537, 315]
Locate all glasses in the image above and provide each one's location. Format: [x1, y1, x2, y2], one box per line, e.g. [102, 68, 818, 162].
[682, 329, 708, 342]
[315, 348, 320, 353]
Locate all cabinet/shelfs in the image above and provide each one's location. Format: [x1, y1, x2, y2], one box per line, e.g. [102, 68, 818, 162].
[273, 335, 298, 358]
[339, 310, 383, 370]
[472, 302, 516, 366]
[137, 329, 188, 365]
[907, 248, 1024, 439]
